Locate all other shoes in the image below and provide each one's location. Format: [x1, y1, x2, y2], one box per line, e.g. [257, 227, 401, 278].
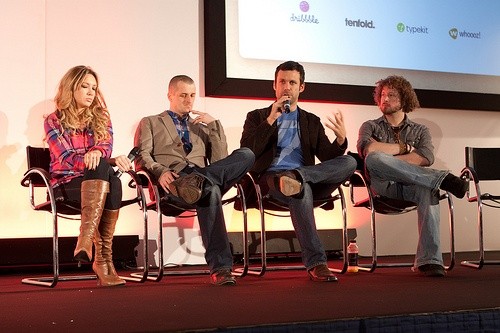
[444, 172, 469, 200]
[417, 262, 444, 277]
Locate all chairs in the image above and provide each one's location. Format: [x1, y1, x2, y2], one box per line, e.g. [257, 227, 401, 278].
[129, 148, 249, 283]
[21, 142, 149, 289]
[234, 152, 348, 277]
[458, 144, 500, 270]
[342, 150, 457, 274]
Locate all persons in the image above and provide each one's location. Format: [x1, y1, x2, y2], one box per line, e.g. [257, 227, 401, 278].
[240, 61, 357, 283]
[356, 76, 468, 278]
[44, 65, 133, 287]
[133, 75, 255, 286]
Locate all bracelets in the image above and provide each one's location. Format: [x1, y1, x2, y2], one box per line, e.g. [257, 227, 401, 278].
[398, 141, 412, 155]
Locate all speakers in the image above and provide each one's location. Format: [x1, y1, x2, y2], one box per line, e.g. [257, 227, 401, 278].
[0, 234, 139, 276]
[226, 228, 357, 264]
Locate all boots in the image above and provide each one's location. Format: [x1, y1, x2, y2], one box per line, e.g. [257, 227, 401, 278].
[72, 177, 110, 273]
[91, 207, 127, 288]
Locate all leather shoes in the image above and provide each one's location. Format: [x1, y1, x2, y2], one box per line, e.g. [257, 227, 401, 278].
[273, 170, 302, 197]
[209, 268, 238, 287]
[309, 264, 340, 283]
[170, 172, 202, 201]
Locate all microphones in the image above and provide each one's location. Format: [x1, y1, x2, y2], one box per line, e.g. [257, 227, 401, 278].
[116, 147, 140, 178]
[283, 97, 290, 114]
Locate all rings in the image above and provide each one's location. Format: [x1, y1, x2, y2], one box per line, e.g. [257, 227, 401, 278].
[92, 157, 96, 159]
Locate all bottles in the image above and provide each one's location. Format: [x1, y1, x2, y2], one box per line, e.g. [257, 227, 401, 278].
[347, 240, 359, 273]
